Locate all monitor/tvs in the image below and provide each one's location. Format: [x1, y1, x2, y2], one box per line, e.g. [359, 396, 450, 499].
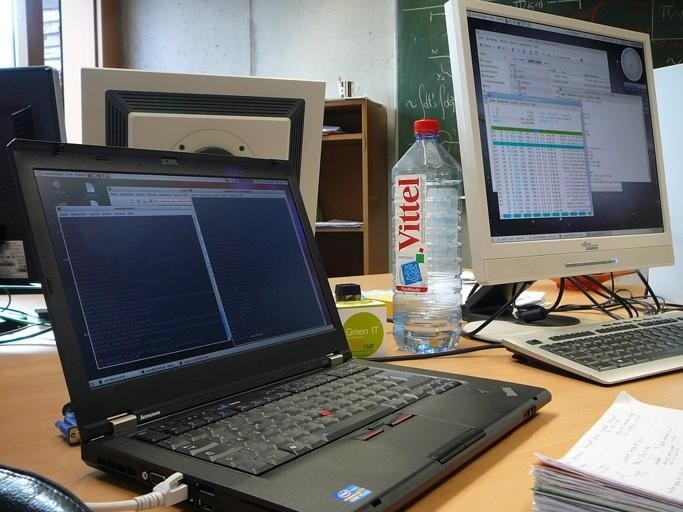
[0, 65, 66, 338]
[441, 0, 683, 345]
[82, 67, 325, 242]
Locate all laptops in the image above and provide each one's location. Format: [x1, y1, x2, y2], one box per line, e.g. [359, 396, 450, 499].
[8, 137, 552, 511]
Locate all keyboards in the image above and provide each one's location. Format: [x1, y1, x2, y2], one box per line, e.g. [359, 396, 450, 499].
[502, 309, 683, 386]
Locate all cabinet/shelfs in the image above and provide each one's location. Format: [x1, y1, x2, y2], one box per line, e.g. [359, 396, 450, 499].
[311, 98, 389, 277]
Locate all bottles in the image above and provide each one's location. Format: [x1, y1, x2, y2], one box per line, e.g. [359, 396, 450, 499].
[390, 119, 461, 354]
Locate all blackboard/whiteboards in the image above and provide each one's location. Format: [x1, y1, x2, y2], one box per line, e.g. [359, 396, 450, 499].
[396, 0, 683, 203]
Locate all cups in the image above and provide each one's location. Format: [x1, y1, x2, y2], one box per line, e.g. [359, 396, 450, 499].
[339, 82, 359, 99]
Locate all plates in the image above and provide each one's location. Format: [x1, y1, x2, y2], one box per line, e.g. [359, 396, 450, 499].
[548, 271, 634, 291]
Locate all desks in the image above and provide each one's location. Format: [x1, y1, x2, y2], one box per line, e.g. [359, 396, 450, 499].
[1, 272, 683, 512]
